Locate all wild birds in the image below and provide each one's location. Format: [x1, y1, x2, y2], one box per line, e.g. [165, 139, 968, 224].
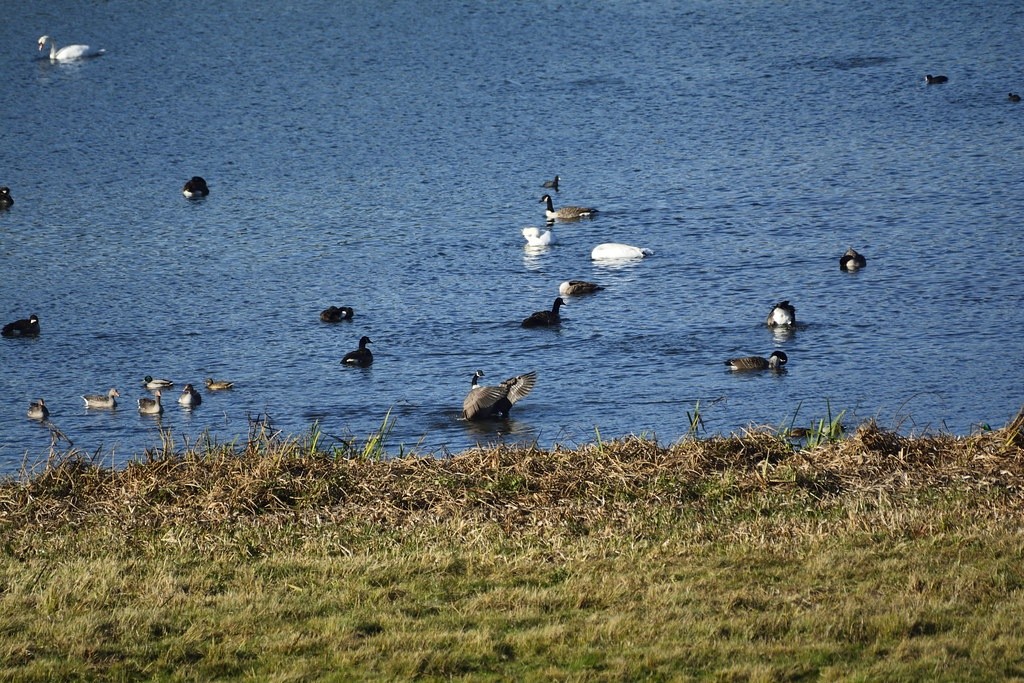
[136, 389, 164, 415]
[522, 227, 558, 245]
[203, 377, 234, 391]
[2, 315, 41, 337]
[177, 384, 202, 405]
[543, 174, 560, 188]
[590, 243, 653, 261]
[520, 297, 566, 325]
[925, 74, 948, 84]
[560, 280, 603, 297]
[462, 369, 538, 420]
[28, 397, 50, 421]
[725, 351, 787, 371]
[539, 193, 599, 219]
[1008, 93, 1020, 101]
[840, 247, 867, 272]
[340, 335, 374, 365]
[320, 306, 355, 322]
[79, 387, 121, 409]
[767, 301, 796, 326]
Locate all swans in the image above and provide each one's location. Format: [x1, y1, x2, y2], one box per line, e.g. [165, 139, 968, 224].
[37, 35, 105, 60]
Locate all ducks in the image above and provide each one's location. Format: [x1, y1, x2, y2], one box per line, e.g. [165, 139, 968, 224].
[184, 176, 209, 200]
[0, 186, 15, 210]
[140, 375, 173, 390]
